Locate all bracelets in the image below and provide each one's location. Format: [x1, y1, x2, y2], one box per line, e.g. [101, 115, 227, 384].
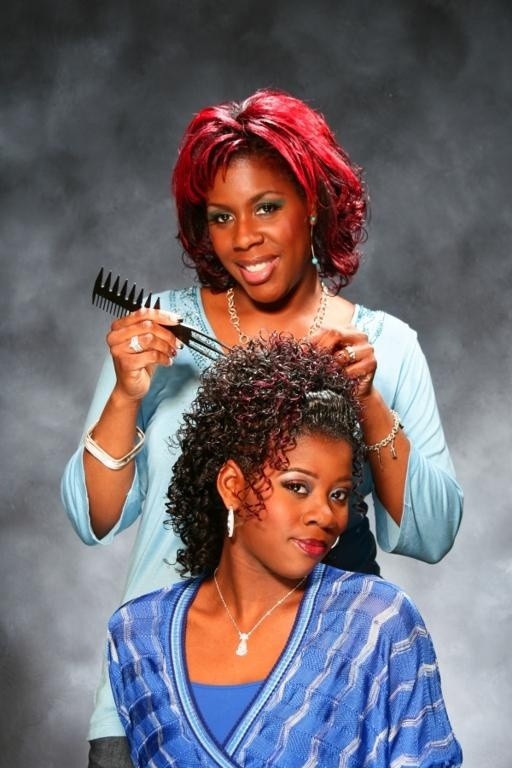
[82, 421, 147, 472]
[361, 409, 405, 471]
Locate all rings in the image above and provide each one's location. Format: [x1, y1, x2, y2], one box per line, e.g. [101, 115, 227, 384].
[128, 334, 143, 352]
[344, 345, 358, 365]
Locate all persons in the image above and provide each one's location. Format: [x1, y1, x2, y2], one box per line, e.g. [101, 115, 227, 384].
[59, 92, 464, 767]
[104, 336, 463, 767]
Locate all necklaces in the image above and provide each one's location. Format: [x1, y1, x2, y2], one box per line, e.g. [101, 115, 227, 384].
[224, 287, 328, 346]
[212, 566, 309, 657]
